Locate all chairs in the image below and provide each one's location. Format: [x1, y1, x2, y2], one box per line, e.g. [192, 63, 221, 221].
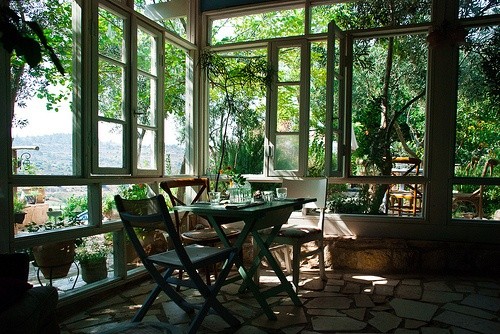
[382, 156, 421, 216]
[252, 176, 329, 296]
[452, 159, 499, 219]
[114, 194, 245, 334]
[158, 177, 245, 294]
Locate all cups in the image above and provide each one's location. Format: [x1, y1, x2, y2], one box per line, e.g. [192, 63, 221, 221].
[263, 191, 274, 205]
[209, 191, 220, 205]
[276, 188, 287, 200]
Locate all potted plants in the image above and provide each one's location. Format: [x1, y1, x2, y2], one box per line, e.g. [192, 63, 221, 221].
[110, 180, 151, 268]
[26, 219, 78, 280]
[13, 190, 28, 226]
[74, 249, 109, 283]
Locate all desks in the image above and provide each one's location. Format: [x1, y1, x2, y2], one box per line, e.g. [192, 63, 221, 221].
[173, 197, 317, 321]
